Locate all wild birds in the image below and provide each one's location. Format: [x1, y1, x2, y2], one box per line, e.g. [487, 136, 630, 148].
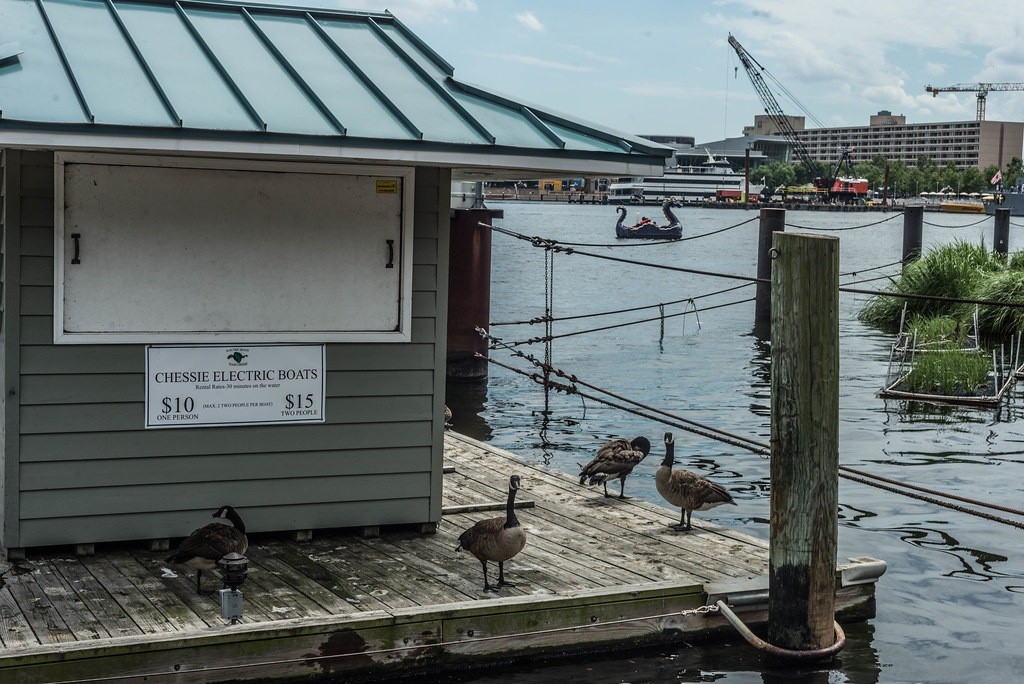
[455, 475, 527, 593]
[164, 505, 249, 595]
[579, 436, 651, 499]
[656, 432, 739, 532]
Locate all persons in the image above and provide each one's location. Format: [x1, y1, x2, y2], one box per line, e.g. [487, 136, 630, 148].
[732, 181, 886, 207]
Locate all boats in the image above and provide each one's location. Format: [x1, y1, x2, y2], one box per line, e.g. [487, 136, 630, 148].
[615, 197, 683, 242]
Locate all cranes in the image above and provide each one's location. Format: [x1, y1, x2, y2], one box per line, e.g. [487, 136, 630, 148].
[925, 81, 1024, 121]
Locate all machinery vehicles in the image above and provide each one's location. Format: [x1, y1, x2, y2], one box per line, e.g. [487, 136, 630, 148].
[726, 34, 869, 206]
